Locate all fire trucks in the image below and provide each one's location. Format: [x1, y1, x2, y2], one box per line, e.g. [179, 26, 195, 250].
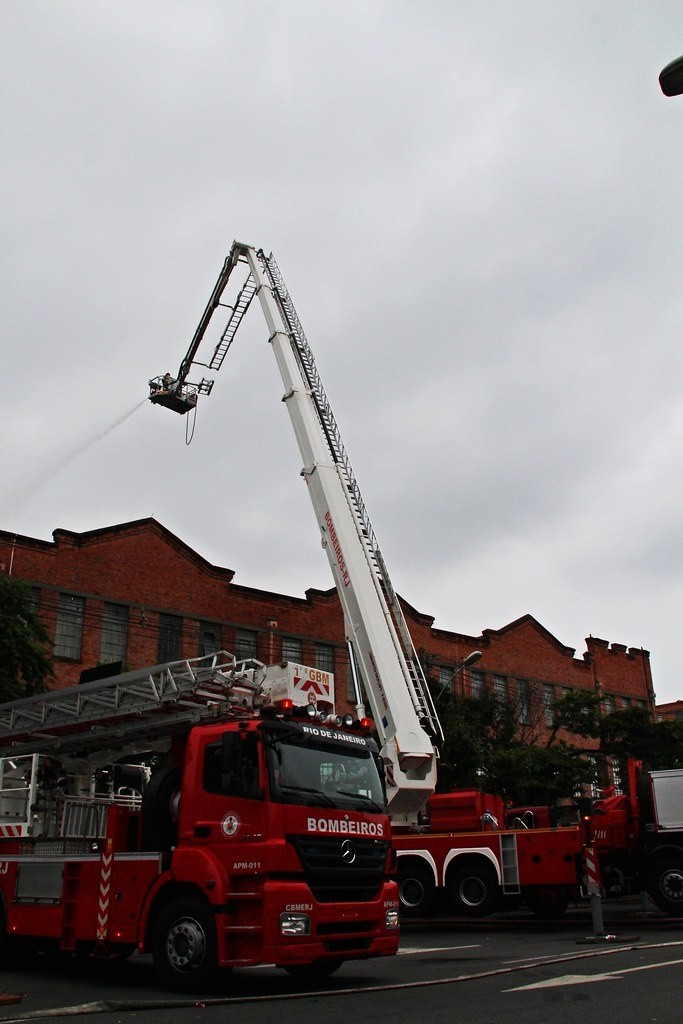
[148, 236, 683, 916]
[0, 648, 401, 993]
